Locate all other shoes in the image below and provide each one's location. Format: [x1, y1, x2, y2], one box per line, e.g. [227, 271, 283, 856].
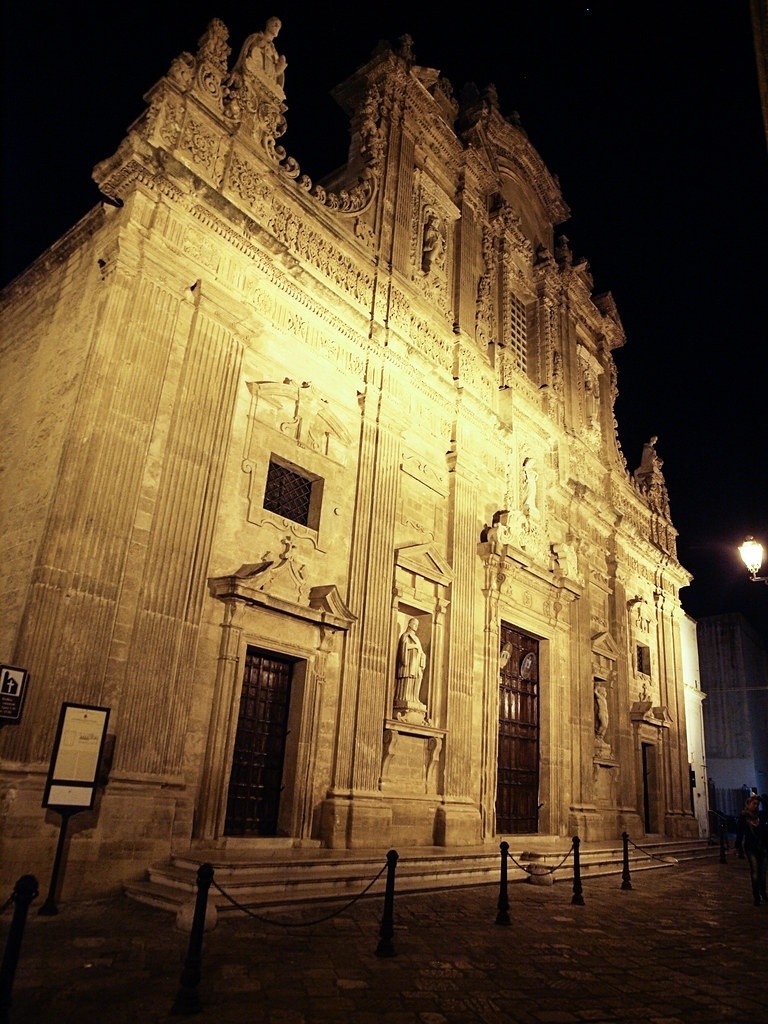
[761, 891, 767, 902]
[753, 896, 760, 906]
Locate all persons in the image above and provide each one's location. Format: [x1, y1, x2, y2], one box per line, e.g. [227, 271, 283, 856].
[641, 436, 658, 470]
[396, 618, 427, 701]
[242, 16, 288, 99]
[595, 686, 608, 743]
[522, 458, 538, 507]
[734, 792, 768, 905]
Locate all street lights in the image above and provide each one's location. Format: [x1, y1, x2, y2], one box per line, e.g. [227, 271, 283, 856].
[737, 535, 768, 774]
